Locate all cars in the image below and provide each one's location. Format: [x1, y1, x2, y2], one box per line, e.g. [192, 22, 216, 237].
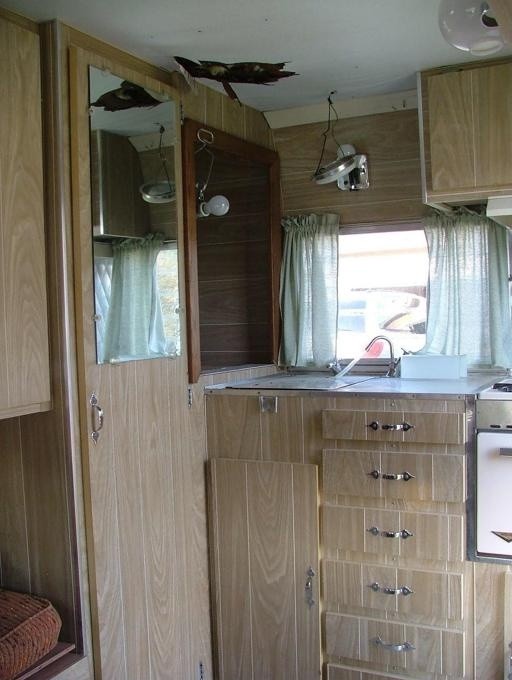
[333, 290, 428, 359]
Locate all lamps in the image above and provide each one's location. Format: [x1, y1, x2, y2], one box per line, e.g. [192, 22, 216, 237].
[195, 128, 229, 218]
[438, 0, 505, 56]
[140, 125, 176, 204]
[310, 90, 369, 192]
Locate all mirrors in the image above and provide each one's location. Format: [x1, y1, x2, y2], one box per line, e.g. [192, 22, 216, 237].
[88, 64, 182, 366]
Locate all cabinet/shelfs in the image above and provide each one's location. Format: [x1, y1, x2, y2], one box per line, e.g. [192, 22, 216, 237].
[208, 398, 505, 680]
[0, 8, 52, 421]
[0, 21, 215, 680]
[90, 129, 150, 259]
[417, 55, 512, 213]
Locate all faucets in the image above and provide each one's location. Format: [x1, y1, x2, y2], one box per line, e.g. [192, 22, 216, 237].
[326, 361, 342, 374]
[365, 336, 400, 378]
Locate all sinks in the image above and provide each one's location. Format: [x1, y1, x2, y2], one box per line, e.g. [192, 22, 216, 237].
[248, 372, 342, 388]
